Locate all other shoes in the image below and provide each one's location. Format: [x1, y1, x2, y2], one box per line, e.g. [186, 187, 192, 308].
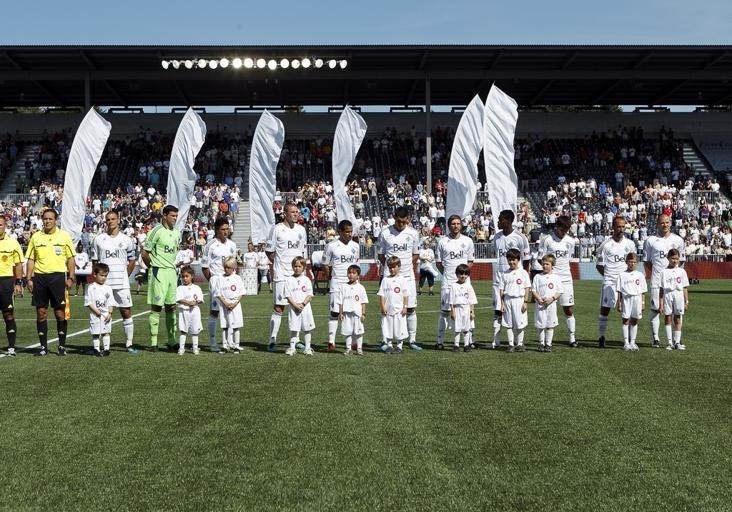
[491, 336, 687, 352]
[268, 338, 474, 355]
[7, 340, 242, 356]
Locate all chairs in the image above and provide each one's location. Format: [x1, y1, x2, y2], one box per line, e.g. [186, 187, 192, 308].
[347, 143, 448, 246]
[57, 149, 235, 198]
[469, 148, 497, 246]
[284, 144, 338, 247]
[515, 142, 697, 246]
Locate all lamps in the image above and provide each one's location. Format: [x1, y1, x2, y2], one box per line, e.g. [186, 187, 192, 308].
[159, 56, 349, 72]
